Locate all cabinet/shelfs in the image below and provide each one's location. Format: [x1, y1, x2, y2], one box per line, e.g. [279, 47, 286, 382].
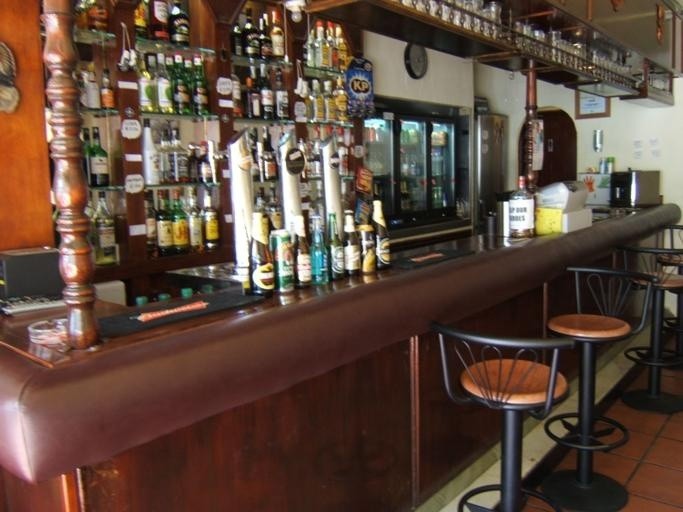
[39, 0, 364, 282]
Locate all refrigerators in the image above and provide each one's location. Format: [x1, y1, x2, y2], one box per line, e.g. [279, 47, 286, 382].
[430, 112, 459, 224]
[356, 107, 399, 236]
[474, 114, 508, 224]
[399, 109, 430, 230]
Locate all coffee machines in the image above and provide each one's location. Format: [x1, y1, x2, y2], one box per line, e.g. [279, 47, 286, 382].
[609, 172, 631, 209]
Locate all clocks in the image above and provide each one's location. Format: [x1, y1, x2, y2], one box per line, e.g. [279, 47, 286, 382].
[405, 43, 428, 79]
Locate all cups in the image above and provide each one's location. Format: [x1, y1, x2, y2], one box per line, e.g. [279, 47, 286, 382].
[405, 1, 632, 89]
[481, 212, 498, 236]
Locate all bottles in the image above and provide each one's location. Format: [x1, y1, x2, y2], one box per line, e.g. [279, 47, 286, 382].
[508, 174, 535, 238]
[372, 179, 386, 220]
[607, 156, 615, 174]
[273, 273, 380, 308]
[431, 147, 449, 210]
[232, 8, 392, 295]
[134, 287, 219, 305]
[134, 0, 217, 257]
[51, 59, 116, 266]
[400, 151, 423, 215]
[598, 158, 606, 174]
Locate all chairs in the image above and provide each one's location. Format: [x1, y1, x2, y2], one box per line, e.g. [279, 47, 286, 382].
[428, 319, 577, 512]
[540, 265, 661, 511]
[655, 225, 683, 371]
[614, 243, 683, 413]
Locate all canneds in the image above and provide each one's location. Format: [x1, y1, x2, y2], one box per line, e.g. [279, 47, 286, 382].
[269, 229, 296, 293]
